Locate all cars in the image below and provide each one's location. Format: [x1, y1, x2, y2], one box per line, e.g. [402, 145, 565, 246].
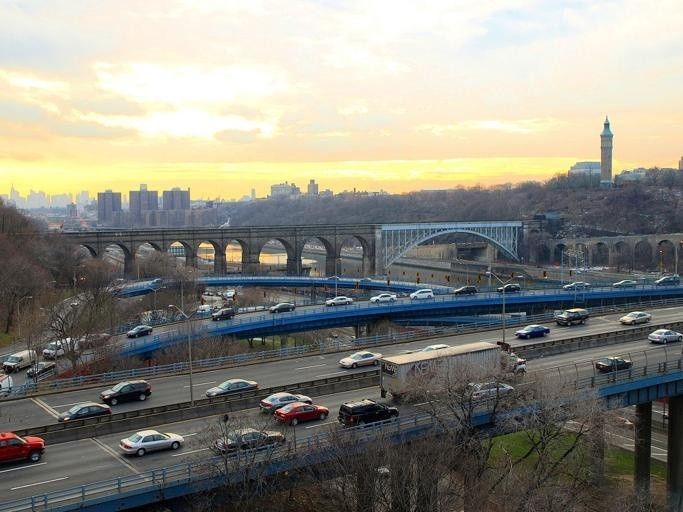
[609, 279, 637, 290]
[328, 328, 340, 339]
[114, 391, 334, 472]
[0, 273, 246, 398]
[562, 282, 592, 291]
[359, 278, 370, 282]
[369, 292, 397, 303]
[514, 323, 551, 340]
[617, 310, 651, 324]
[204, 377, 258, 398]
[646, 328, 682, 344]
[328, 276, 339, 280]
[323, 295, 353, 306]
[53, 403, 112, 423]
[350, 334, 357, 342]
[591, 353, 634, 373]
[269, 301, 295, 314]
[422, 344, 449, 352]
[337, 349, 383, 369]
[374, 462, 390, 479]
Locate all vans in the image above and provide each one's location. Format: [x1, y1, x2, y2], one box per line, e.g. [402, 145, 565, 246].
[654, 276, 681, 286]
[408, 287, 435, 299]
[462, 380, 515, 402]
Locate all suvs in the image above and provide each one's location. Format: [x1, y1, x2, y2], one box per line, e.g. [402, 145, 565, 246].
[0, 431, 47, 466]
[497, 283, 521, 293]
[554, 306, 590, 328]
[452, 285, 480, 295]
[335, 394, 400, 430]
[99, 379, 152, 406]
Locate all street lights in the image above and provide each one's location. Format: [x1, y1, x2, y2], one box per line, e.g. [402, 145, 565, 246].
[486, 268, 523, 340]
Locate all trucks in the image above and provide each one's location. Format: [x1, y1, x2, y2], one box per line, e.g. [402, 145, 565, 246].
[379, 343, 528, 405]
